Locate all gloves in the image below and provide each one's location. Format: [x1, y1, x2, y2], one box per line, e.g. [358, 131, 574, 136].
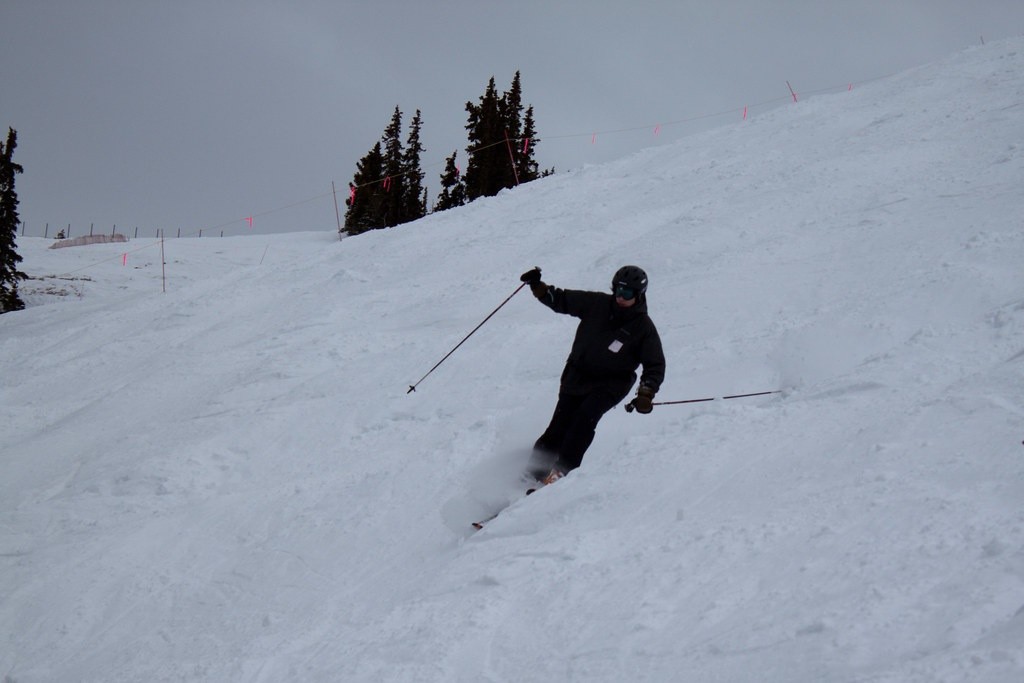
[625, 394, 655, 414]
[520, 269, 546, 297]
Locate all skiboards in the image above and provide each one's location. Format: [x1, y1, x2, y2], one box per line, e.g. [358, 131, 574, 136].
[472, 485, 551, 530]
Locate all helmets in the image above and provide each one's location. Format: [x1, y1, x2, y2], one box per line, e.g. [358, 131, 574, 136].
[612, 266, 648, 295]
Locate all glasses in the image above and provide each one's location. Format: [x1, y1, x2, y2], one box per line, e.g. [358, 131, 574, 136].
[611, 286, 637, 300]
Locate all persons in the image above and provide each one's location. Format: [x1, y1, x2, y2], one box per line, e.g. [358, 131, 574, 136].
[520, 265, 668, 492]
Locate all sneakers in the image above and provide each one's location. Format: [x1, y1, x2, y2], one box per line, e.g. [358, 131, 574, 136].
[541, 466, 567, 486]
